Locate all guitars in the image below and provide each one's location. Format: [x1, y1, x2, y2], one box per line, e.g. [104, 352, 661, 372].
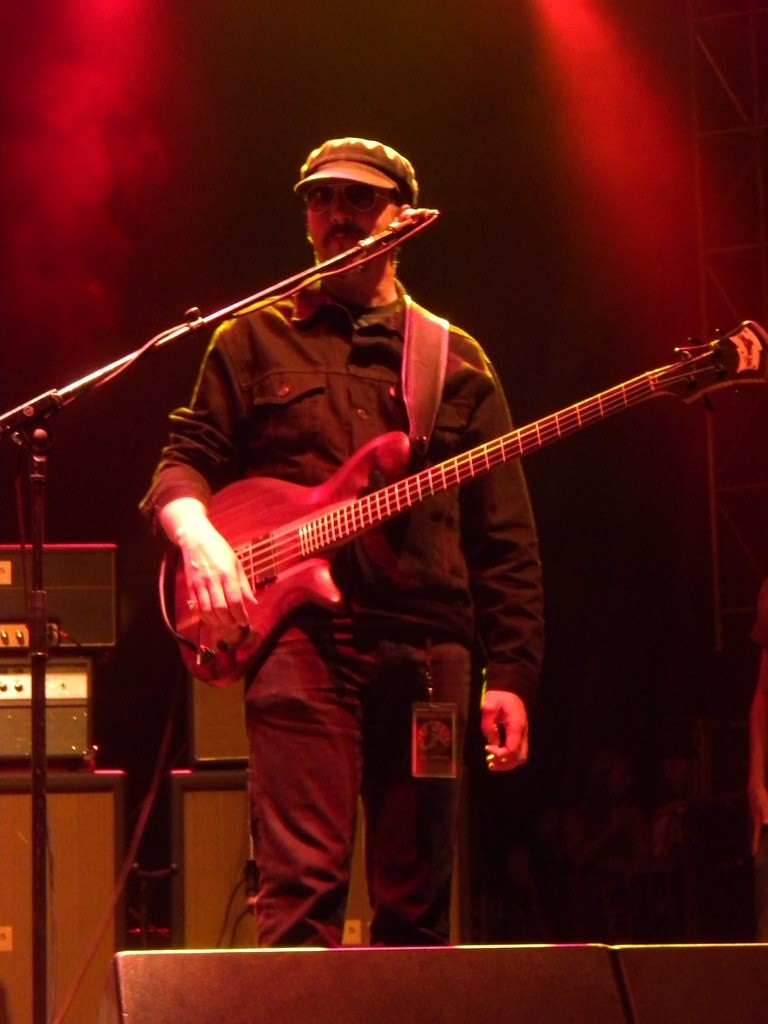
[158, 319, 768, 692]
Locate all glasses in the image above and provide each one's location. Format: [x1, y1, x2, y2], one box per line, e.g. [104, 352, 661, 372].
[303, 182, 396, 211]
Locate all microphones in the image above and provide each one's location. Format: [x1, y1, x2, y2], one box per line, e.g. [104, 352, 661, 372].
[398, 208, 440, 226]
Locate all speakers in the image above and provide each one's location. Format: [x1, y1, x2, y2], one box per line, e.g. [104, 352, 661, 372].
[170, 767, 372, 951]
[188, 670, 251, 767]
[55, 943, 627, 1024]
[612, 943, 768, 1024]
[0, 764, 126, 1024]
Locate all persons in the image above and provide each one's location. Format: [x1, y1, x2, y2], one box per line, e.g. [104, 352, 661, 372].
[749, 578, 768, 855]
[138, 138, 537, 947]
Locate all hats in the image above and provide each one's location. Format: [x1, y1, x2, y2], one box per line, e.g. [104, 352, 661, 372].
[293, 137, 418, 209]
